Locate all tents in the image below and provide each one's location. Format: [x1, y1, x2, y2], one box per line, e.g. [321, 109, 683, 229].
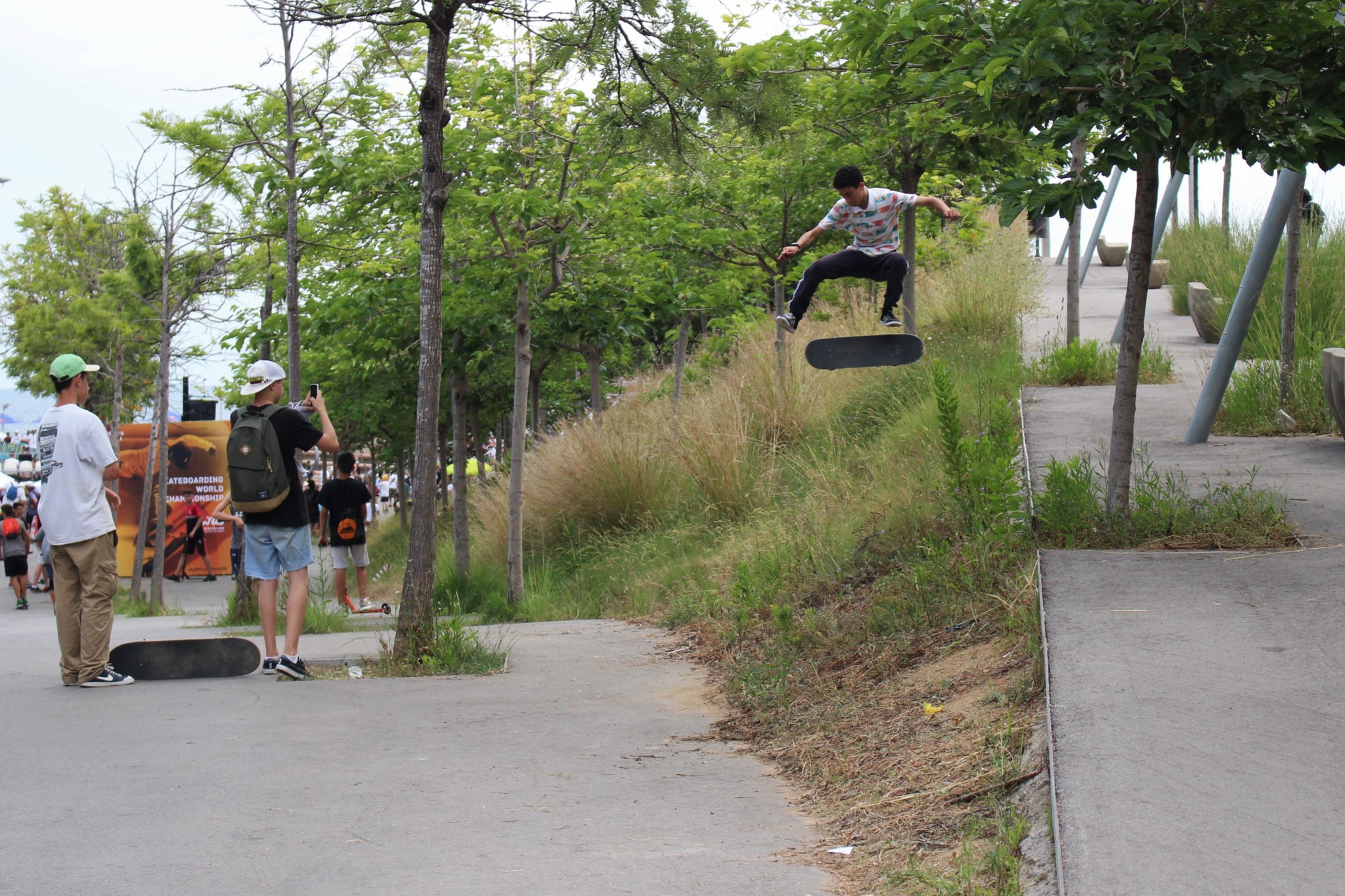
[442, 453, 492, 478]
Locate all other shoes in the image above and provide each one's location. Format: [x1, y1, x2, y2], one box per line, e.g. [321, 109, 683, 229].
[9, 583, 14, 588]
[26, 581, 33, 588]
[165, 573, 180, 582]
[203, 574, 217, 582]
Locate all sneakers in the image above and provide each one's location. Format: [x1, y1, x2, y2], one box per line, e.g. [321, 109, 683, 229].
[81, 666, 134, 687]
[262, 657, 277, 674]
[359, 598, 374, 610]
[31, 586, 42, 592]
[275, 655, 314, 680]
[775, 312, 798, 334]
[880, 307, 902, 327]
[15, 598, 29, 609]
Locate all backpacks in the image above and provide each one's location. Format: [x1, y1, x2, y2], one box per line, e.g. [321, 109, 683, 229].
[6, 481, 21, 500]
[226, 405, 290, 512]
[2, 517, 20, 539]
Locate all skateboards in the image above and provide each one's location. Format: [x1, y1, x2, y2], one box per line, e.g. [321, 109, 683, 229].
[803, 332, 926, 372]
[102, 637, 262, 681]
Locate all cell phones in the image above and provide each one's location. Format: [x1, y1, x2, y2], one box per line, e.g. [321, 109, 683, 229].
[310, 384, 319, 399]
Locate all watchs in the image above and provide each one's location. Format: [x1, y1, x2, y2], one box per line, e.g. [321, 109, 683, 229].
[791, 243, 801, 252]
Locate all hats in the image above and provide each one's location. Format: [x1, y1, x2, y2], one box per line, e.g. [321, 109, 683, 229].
[49, 354, 101, 382]
[240, 359, 286, 395]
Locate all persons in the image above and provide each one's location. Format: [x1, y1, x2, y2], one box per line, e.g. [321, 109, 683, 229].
[34, 354, 134, 687]
[0, 427, 457, 618]
[774, 167, 961, 334]
[226, 360, 338, 680]
